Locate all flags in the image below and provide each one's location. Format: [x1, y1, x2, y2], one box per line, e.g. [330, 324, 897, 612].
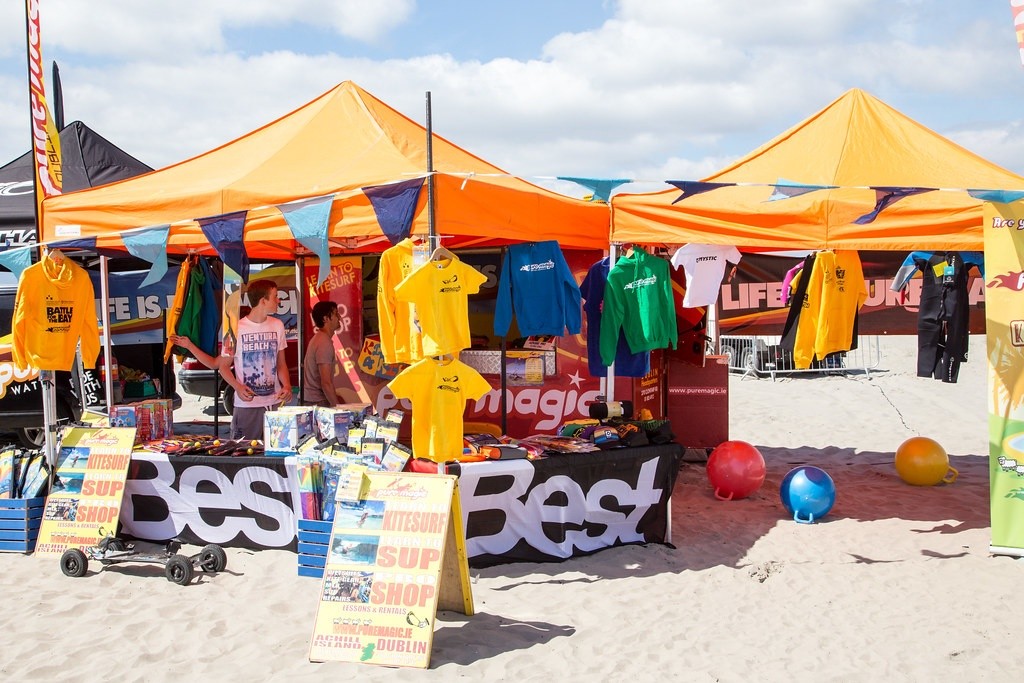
[26, 0, 62, 258]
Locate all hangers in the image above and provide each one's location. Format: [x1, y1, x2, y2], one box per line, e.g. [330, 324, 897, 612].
[46, 247, 66, 260]
[614, 242, 634, 258]
[184, 248, 217, 262]
[430, 353, 454, 366]
[408, 234, 459, 264]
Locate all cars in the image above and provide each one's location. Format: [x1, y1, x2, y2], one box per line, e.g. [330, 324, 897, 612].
[178, 340, 301, 417]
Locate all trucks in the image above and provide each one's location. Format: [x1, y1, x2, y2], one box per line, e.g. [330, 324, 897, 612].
[719, 335, 847, 377]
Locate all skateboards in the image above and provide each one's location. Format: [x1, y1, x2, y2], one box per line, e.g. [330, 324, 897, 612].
[60, 537, 228, 587]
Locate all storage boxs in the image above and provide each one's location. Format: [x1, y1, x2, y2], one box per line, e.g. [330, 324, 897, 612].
[262, 403, 373, 457]
[0, 497, 44, 553]
[296, 519, 333, 577]
[97, 355, 172, 445]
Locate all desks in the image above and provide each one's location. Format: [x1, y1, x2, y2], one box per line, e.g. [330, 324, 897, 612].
[115, 439, 299, 554]
[444, 439, 686, 570]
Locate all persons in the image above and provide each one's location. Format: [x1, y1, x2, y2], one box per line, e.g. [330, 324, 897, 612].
[298, 301, 346, 407]
[170, 334, 221, 369]
[219, 280, 292, 441]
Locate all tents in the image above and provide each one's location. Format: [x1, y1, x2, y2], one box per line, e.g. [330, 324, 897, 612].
[0, 81, 1024, 465]
